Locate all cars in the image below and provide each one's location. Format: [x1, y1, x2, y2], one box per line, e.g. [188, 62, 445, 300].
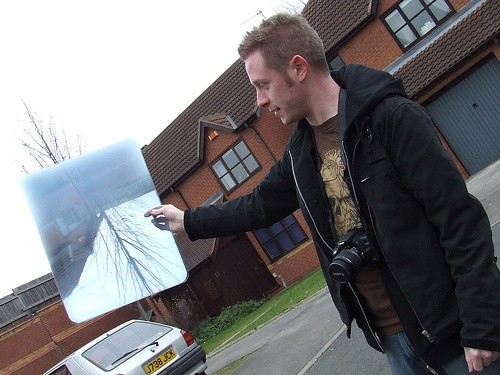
[42, 318, 209, 375]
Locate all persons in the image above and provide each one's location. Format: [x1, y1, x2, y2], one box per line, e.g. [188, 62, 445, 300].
[143, 12, 500, 375]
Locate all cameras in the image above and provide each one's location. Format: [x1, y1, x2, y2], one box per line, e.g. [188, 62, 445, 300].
[327, 227, 380, 283]
